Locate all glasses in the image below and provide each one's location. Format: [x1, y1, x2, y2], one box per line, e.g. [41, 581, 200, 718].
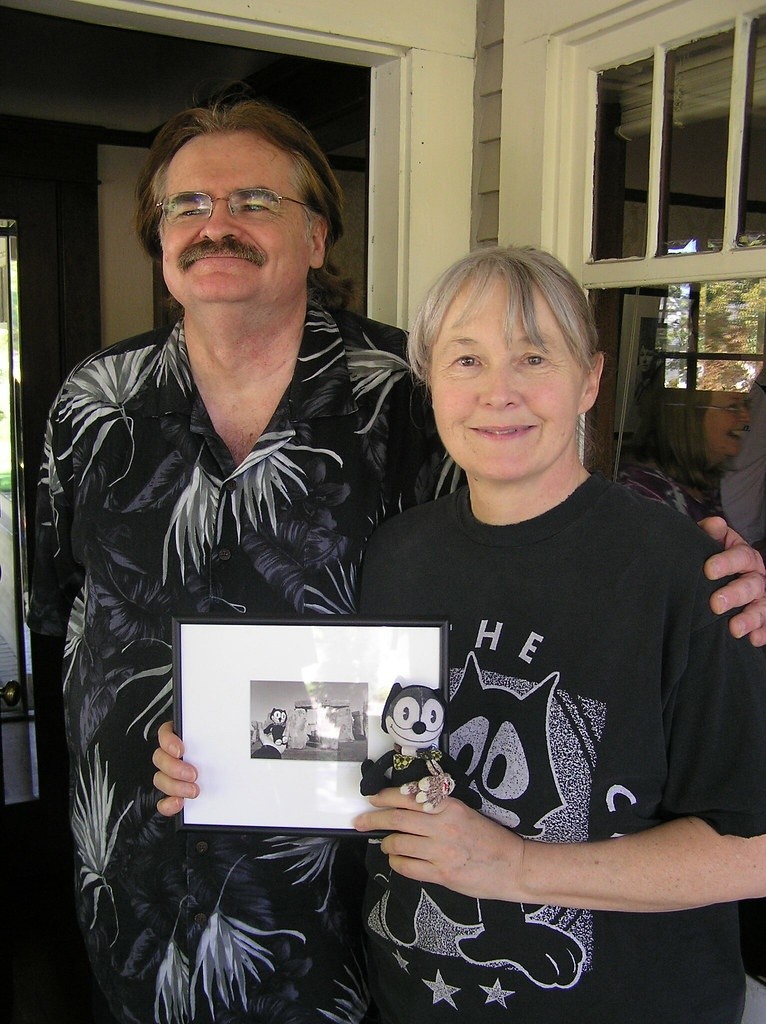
[668, 402, 752, 416]
[155, 187, 324, 228]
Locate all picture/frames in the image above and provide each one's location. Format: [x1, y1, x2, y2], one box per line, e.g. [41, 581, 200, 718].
[612, 287, 697, 438]
[171, 614, 446, 837]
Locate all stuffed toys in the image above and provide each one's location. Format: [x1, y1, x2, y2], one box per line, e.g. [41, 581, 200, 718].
[262, 707, 290, 746]
[361, 682, 467, 811]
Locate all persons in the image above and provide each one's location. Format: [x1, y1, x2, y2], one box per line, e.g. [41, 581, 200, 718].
[250, 708, 289, 759]
[27, 100, 765, 1024]
